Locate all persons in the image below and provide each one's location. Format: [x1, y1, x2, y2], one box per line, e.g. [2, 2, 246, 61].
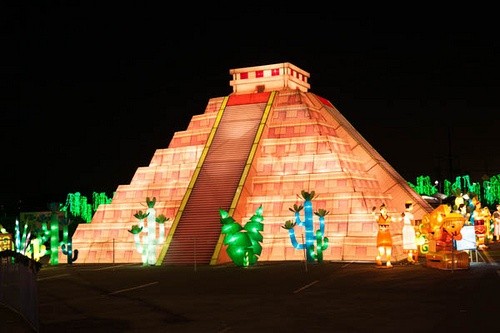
[372, 203, 406, 267]
[401, 201, 420, 264]
[455, 194, 500, 251]
